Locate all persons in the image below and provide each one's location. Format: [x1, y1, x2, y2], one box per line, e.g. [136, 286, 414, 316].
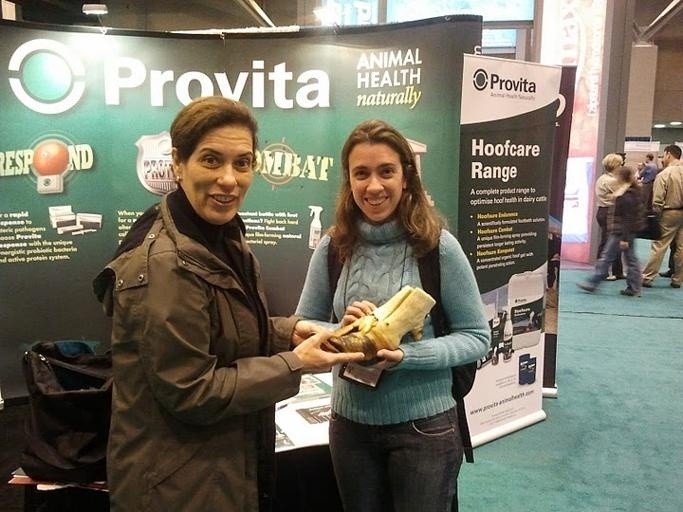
[93, 96, 367, 511]
[575, 145, 682, 298]
[293, 120, 492, 512]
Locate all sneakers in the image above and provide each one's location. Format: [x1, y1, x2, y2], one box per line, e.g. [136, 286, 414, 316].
[576, 268, 680, 296]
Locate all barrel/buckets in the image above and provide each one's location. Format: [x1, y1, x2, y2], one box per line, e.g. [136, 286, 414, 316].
[505, 270, 545, 351]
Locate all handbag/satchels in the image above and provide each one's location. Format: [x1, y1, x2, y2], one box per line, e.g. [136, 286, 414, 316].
[20, 339, 111, 483]
[636, 210, 661, 240]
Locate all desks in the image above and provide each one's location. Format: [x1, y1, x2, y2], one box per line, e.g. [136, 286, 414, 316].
[19, 362, 458, 510]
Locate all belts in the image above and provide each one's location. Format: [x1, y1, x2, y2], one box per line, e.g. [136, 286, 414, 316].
[662, 207, 682, 209]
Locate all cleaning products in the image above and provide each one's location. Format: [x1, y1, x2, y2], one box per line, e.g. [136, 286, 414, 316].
[307, 205, 325, 250]
